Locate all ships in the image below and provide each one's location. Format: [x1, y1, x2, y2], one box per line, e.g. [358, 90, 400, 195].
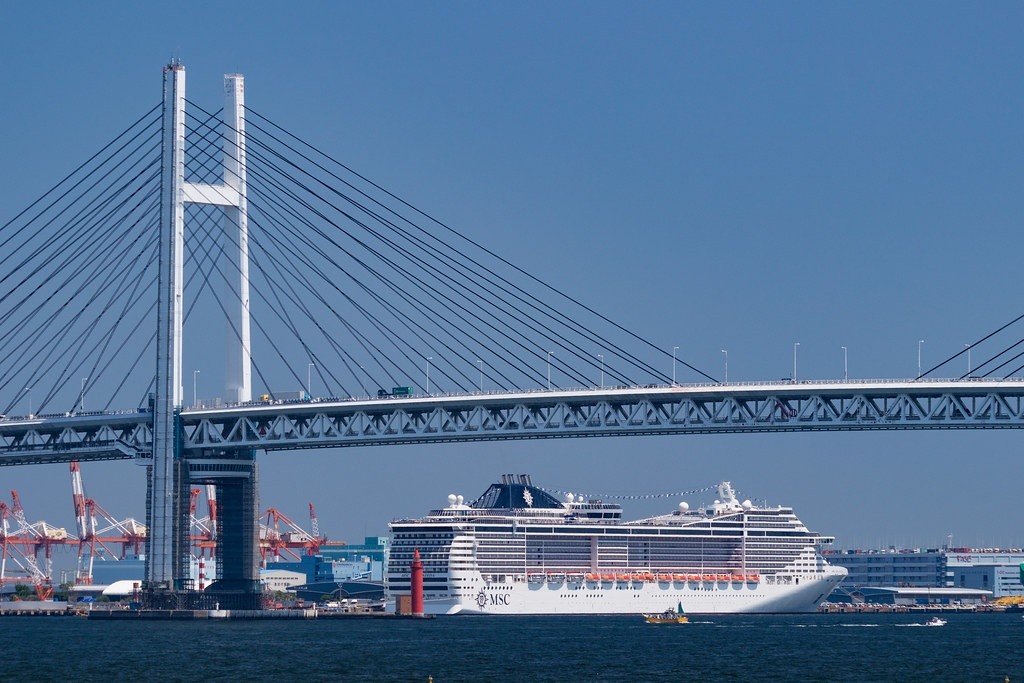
[383, 473, 852, 614]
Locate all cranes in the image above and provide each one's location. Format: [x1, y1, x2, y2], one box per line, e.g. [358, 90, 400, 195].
[0, 460, 348, 602]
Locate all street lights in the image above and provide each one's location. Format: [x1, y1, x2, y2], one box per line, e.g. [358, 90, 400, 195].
[597, 353, 604, 389]
[721, 350, 728, 385]
[793, 342, 801, 382]
[81, 378, 88, 411]
[476, 360, 483, 394]
[964, 344, 971, 379]
[25, 387, 32, 416]
[548, 351, 554, 390]
[426, 357, 434, 395]
[193, 370, 200, 407]
[308, 364, 315, 397]
[841, 347, 847, 382]
[672, 346, 680, 386]
[918, 340, 926, 382]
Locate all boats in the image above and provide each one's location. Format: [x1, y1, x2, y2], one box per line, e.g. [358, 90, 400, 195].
[656, 575, 672, 584]
[703, 575, 715, 584]
[617, 575, 629, 583]
[732, 576, 744, 584]
[924, 616, 948, 627]
[717, 575, 730, 584]
[674, 576, 686, 584]
[687, 575, 702, 584]
[632, 575, 645, 583]
[642, 608, 689, 624]
[600, 573, 615, 584]
[586, 573, 600, 583]
[745, 576, 759, 584]
